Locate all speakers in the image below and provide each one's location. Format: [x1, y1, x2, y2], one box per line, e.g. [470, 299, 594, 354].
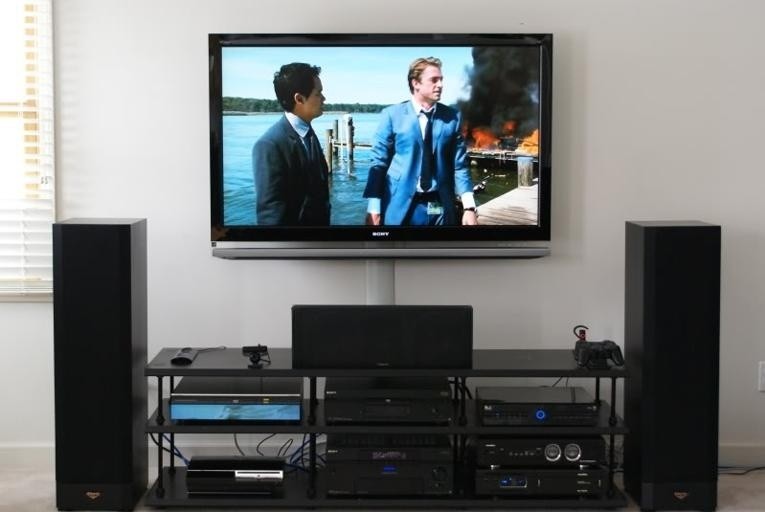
[47, 214, 149, 511]
[621, 218, 723, 512]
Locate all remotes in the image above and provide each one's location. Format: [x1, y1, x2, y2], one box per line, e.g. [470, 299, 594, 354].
[170, 345, 200, 367]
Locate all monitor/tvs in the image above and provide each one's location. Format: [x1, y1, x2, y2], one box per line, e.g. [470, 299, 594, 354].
[205, 31, 556, 259]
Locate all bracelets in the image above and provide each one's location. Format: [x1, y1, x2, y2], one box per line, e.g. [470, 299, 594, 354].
[462, 206, 478, 212]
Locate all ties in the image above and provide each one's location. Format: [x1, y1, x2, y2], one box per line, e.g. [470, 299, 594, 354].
[307, 131, 321, 168]
[421, 108, 439, 190]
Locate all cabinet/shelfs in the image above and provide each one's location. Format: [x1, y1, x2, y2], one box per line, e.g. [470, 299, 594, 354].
[142, 348, 628, 511]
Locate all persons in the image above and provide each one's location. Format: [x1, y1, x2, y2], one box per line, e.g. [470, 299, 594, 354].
[361, 57, 479, 227]
[252, 62, 331, 227]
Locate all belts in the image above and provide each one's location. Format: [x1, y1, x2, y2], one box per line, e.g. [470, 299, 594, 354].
[414, 191, 439, 204]
